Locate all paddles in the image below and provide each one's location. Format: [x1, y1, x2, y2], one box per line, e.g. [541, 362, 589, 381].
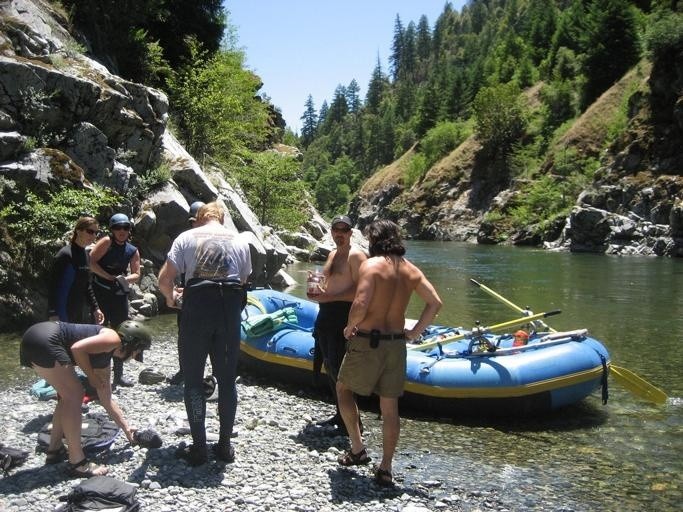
[470, 278, 667, 404]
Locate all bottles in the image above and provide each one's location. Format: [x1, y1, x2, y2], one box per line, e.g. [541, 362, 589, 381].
[306, 273, 326, 301]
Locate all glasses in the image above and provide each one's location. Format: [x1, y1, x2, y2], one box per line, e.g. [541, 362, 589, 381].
[84, 227, 99, 236]
[114, 224, 129, 231]
[334, 228, 349, 233]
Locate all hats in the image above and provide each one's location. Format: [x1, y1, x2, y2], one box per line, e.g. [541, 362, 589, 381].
[332, 214, 352, 227]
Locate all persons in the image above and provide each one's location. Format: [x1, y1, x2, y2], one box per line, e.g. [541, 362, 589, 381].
[51, 218, 104, 325]
[88, 213, 141, 387]
[315, 216, 368, 437]
[158, 201, 252, 466]
[336, 218, 443, 484]
[170, 200, 206, 385]
[20, 320, 152, 476]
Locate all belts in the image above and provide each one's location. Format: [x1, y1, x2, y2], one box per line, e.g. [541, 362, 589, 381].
[356, 331, 406, 340]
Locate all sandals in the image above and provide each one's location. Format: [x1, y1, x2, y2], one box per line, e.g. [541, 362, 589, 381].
[66, 458, 108, 476]
[338, 447, 372, 466]
[45, 445, 68, 463]
[375, 468, 393, 487]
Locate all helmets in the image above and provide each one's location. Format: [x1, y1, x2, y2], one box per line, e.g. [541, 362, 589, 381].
[108, 213, 130, 228]
[189, 201, 206, 221]
[117, 320, 151, 351]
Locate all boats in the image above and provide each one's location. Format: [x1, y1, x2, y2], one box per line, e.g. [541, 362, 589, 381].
[237, 288, 611, 415]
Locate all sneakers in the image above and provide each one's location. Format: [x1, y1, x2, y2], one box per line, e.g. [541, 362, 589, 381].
[179, 444, 207, 463]
[322, 413, 364, 436]
[216, 442, 235, 462]
